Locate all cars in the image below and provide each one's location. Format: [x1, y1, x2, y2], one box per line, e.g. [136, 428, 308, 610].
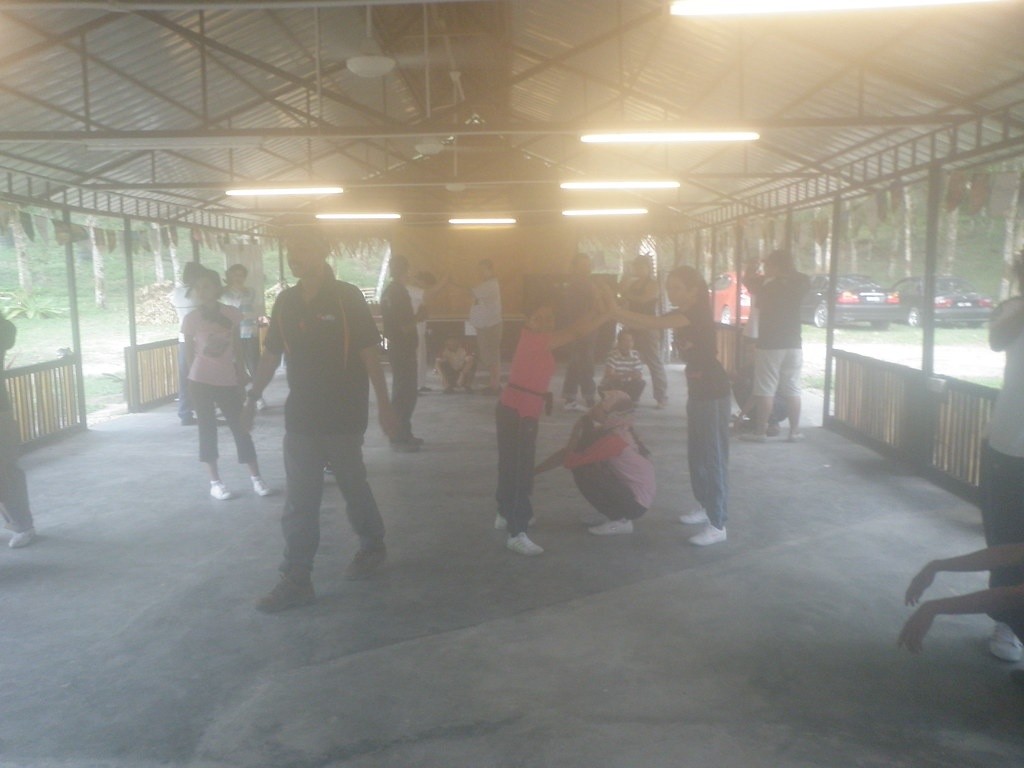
[794, 274, 899, 330]
[704, 272, 752, 325]
[889, 277, 994, 328]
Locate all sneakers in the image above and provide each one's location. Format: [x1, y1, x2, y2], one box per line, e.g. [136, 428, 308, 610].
[8, 527, 36, 549]
[210, 481, 231, 500]
[506, 531, 544, 556]
[678, 503, 711, 524]
[988, 621, 1024, 662]
[494, 513, 535, 529]
[689, 525, 728, 546]
[250, 476, 272, 496]
[587, 519, 633, 536]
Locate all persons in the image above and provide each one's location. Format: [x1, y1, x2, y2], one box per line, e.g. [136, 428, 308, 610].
[169, 261, 266, 428]
[381, 250, 818, 455]
[249, 231, 404, 615]
[978, 249, 1024, 661]
[898, 543, 1024, 654]
[1, 314, 37, 549]
[491, 283, 622, 555]
[533, 390, 656, 535]
[179, 269, 271, 501]
[586, 265, 734, 546]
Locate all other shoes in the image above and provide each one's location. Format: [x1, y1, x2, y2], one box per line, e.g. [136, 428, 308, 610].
[788, 433, 805, 442]
[736, 433, 767, 441]
[657, 398, 675, 410]
[407, 435, 424, 444]
[335, 551, 389, 581]
[250, 581, 315, 613]
[388, 440, 420, 453]
